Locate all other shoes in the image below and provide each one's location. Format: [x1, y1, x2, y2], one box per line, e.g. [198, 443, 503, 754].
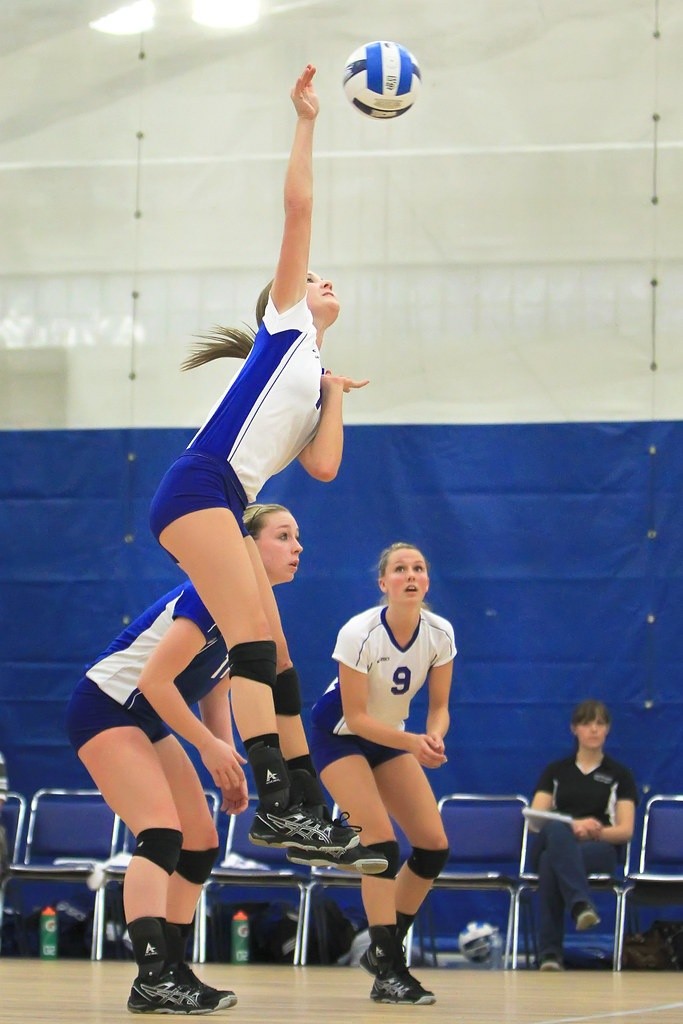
[541, 955, 563, 971]
[574, 905, 601, 929]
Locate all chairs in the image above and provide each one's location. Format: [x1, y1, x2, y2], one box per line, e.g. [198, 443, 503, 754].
[300, 801, 405, 967]
[404, 792, 527, 971]
[197, 792, 306, 968]
[0, 790, 120, 961]
[94, 789, 220, 964]
[617, 793, 683, 975]
[511, 784, 633, 973]
[0, 792, 27, 865]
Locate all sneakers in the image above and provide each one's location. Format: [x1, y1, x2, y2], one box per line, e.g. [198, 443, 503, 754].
[247, 803, 359, 852]
[360, 945, 436, 1005]
[127, 964, 237, 1015]
[286, 802, 388, 875]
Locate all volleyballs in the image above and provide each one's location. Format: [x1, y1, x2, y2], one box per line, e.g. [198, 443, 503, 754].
[342, 40, 422, 119]
[458, 921, 501, 963]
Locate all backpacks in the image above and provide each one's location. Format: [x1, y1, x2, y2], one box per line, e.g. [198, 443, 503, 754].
[82, 887, 133, 961]
[267, 901, 350, 962]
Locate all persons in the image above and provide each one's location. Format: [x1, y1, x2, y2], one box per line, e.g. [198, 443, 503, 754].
[530, 700, 639, 972]
[309, 542, 457, 1005]
[148, 64, 388, 873]
[66, 504, 304, 1014]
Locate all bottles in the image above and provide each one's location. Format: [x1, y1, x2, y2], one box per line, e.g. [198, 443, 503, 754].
[40, 906, 58, 960]
[231, 911, 250, 964]
